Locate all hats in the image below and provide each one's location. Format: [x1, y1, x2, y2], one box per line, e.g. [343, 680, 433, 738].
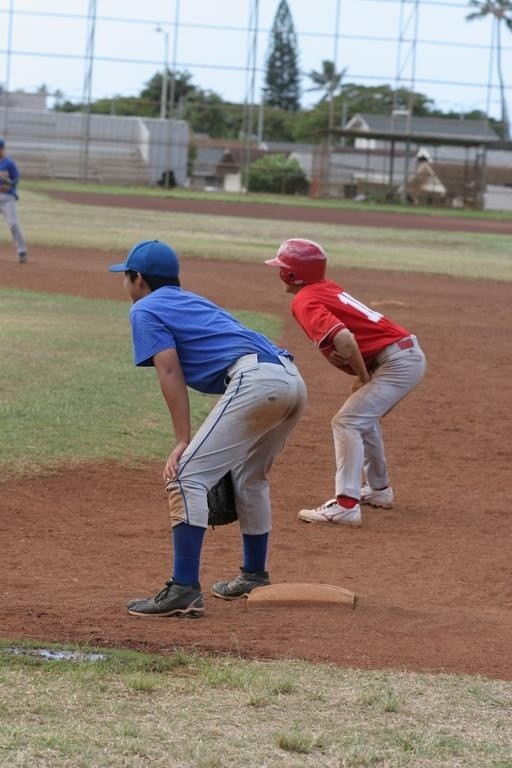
[106, 240, 180, 279]
[0, 138, 5, 148]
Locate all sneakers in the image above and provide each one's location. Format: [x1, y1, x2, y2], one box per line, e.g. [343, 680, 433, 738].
[360, 481, 395, 510]
[297, 498, 363, 528]
[126, 576, 206, 618]
[210, 566, 271, 601]
[18, 251, 27, 264]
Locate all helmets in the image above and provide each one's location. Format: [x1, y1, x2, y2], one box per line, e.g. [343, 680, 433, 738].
[263, 236, 328, 287]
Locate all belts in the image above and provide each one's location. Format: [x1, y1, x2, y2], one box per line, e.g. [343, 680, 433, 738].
[396, 337, 415, 351]
[225, 351, 294, 385]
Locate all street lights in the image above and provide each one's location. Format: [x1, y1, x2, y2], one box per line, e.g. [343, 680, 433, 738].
[153, 24, 170, 120]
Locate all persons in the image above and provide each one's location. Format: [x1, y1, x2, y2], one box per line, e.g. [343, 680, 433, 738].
[107, 240, 308, 618]
[265, 236, 427, 528]
[0, 140, 29, 263]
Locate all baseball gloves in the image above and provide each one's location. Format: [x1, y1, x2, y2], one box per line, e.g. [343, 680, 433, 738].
[206, 468, 238, 531]
